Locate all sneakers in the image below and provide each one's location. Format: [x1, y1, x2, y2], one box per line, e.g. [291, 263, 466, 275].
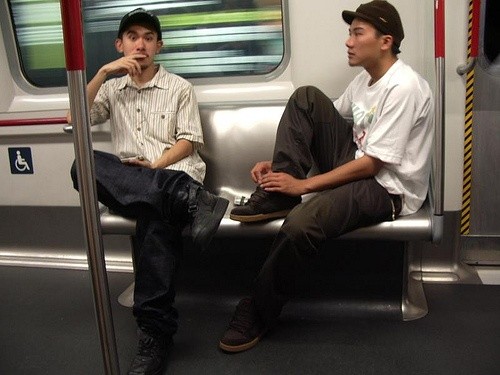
[127, 324, 172, 375]
[216, 300, 280, 351]
[230, 186, 302, 222]
[188, 192, 229, 252]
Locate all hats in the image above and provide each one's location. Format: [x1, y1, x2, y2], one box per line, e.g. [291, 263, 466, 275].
[118, 8, 161, 38]
[342, 0, 404, 47]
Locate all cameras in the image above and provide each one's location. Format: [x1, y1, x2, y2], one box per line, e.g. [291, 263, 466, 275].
[118, 153, 143, 163]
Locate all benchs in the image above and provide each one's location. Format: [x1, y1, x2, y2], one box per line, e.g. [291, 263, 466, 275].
[63, 100, 432, 322]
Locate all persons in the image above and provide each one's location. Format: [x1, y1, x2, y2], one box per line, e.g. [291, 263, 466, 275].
[216, 0, 436, 351]
[67, 8, 230, 375]
